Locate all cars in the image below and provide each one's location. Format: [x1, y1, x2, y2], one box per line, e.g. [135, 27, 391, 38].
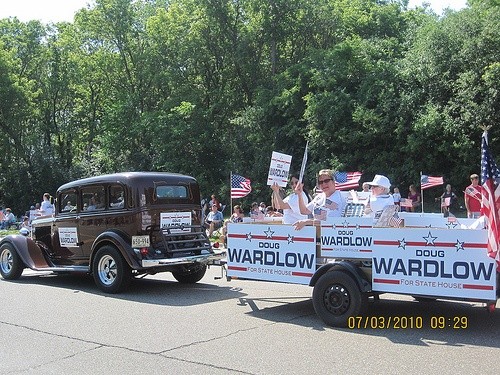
[0, 171, 216, 294]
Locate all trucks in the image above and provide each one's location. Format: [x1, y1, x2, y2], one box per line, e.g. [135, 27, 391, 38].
[223, 211, 500, 328]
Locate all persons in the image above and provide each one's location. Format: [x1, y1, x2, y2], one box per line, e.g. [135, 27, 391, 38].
[64, 201, 73, 211]
[393, 187, 402, 206]
[272, 174, 309, 224]
[201, 194, 223, 238]
[292, 169, 342, 230]
[0, 193, 56, 236]
[361, 181, 370, 193]
[464, 174, 483, 218]
[441, 184, 456, 212]
[349, 174, 394, 217]
[406, 184, 421, 212]
[221, 205, 244, 239]
[251, 200, 282, 219]
[87, 190, 124, 210]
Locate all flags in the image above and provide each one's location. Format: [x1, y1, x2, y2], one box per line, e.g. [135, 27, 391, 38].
[231, 174, 252, 198]
[480, 130, 500, 275]
[421, 173, 443, 190]
[333, 171, 361, 190]
[399, 198, 412, 207]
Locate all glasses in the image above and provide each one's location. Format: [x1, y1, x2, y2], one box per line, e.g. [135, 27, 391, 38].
[319, 178, 333, 185]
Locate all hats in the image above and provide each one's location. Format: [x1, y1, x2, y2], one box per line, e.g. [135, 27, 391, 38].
[367, 175, 391, 189]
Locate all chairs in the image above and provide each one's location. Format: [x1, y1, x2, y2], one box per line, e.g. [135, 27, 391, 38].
[98, 194, 106, 208]
[221, 203, 227, 213]
